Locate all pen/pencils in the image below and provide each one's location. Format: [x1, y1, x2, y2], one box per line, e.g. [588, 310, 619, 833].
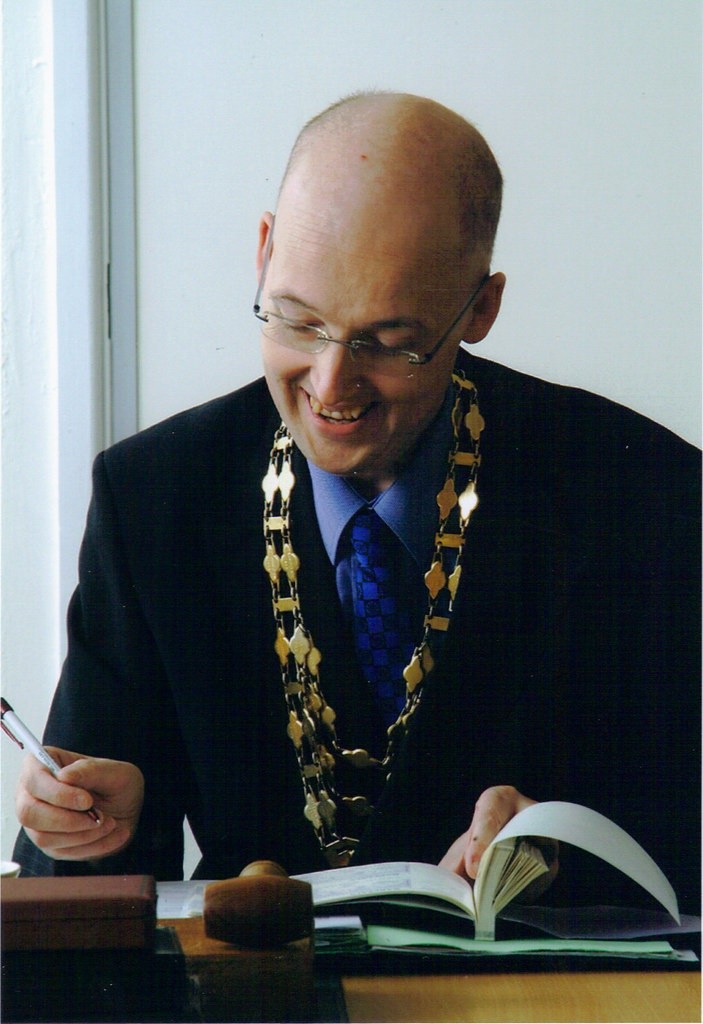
[1, 698, 102, 825]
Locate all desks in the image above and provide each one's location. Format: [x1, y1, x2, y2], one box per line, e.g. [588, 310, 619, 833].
[157, 917, 702, 1023]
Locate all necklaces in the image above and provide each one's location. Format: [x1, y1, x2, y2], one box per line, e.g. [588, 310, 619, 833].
[260, 375, 484, 869]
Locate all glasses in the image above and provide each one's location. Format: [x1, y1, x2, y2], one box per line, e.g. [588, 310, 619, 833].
[253, 216, 488, 377]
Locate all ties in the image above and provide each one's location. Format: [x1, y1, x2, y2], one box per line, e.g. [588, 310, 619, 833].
[350, 508, 427, 724]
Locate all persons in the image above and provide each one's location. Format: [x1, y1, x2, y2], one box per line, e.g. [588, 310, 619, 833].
[10, 93, 703, 918]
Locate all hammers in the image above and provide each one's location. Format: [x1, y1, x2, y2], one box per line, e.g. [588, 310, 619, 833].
[204, 860, 314, 949]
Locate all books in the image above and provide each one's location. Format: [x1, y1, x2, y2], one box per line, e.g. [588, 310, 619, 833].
[289, 802, 701, 970]
